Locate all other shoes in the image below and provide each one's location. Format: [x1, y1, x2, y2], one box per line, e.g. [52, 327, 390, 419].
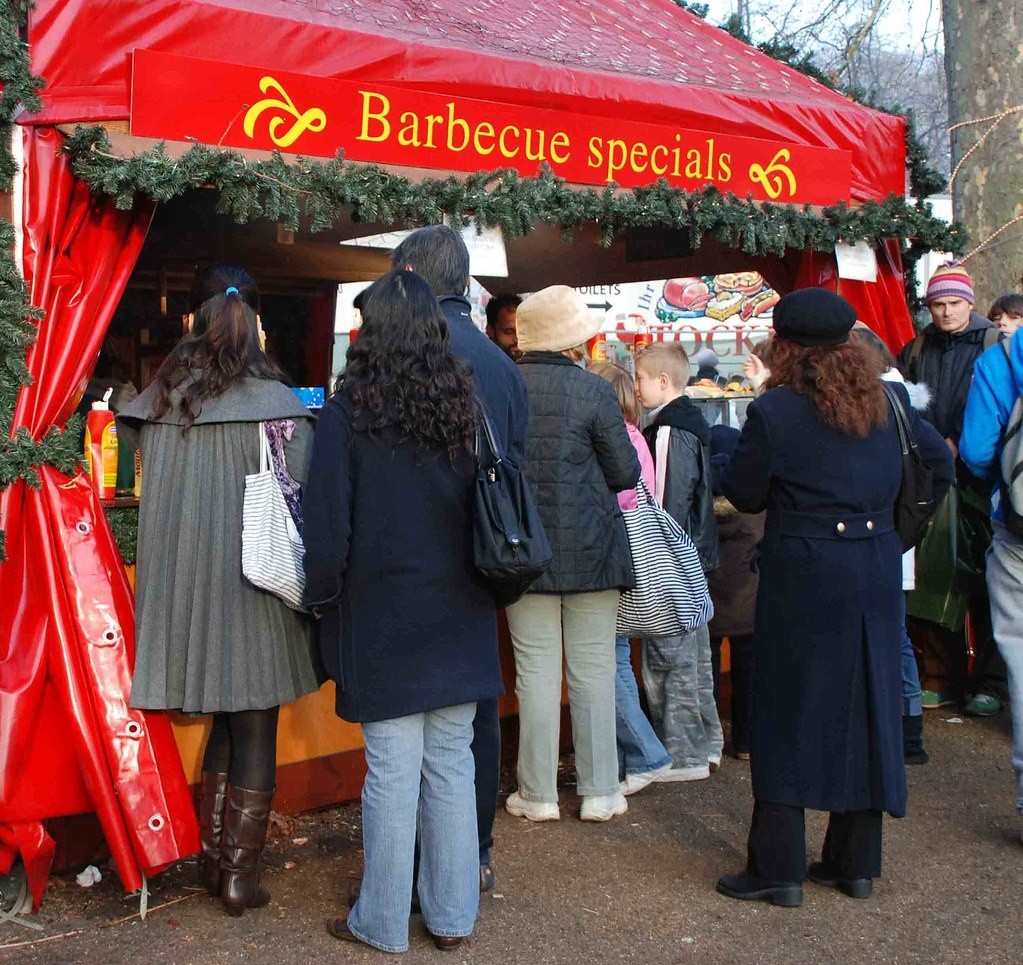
[436, 935, 462, 950]
[479, 865, 495, 892]
[327, 915, 362, 941]
[654, 755, 721, 783]
[903, 718, 929, 764]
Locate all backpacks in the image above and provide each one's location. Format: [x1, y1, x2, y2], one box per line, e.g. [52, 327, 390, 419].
[1000, 397, 1023, 544]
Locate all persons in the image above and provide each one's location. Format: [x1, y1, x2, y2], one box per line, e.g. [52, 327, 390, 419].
[958, 321, 1023, 850]
[491, 261, 1023, 796]
[116, 259, 319, 917]
[704, 285, 955, 909]
[350, 225, 528, 913]
[506, 284, 632, 824]
[303, 270, 501, 951]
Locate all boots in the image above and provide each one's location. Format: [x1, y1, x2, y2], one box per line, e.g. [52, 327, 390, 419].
[196, 771, 276, 920]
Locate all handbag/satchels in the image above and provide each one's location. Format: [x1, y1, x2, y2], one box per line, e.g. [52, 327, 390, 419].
[899, 452, 936, 552]
[469, 457, 551, 605]
[616, 475, 714, 638]
[241, 423, 308, 610]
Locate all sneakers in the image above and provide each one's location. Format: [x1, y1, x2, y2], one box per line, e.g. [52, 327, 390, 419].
[619, 763, 672, 796]
[580, 793, 629, 821]
[505, 791, 561, 823]
[921, 688, 1001, 716]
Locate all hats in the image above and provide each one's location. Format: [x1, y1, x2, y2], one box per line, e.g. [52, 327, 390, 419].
[926, 259, 975, 306]
[773, 287, 858, 346]
[515, 284, 605, 352]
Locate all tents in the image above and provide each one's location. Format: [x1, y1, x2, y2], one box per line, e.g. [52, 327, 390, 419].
[5, 0, 913, 893]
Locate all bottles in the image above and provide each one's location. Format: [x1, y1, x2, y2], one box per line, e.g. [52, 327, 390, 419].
[84, 387, 118, 501]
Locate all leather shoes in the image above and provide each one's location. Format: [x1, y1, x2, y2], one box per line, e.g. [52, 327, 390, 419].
[717, 873, 804, 907]
[809, 862, 873, 899]
